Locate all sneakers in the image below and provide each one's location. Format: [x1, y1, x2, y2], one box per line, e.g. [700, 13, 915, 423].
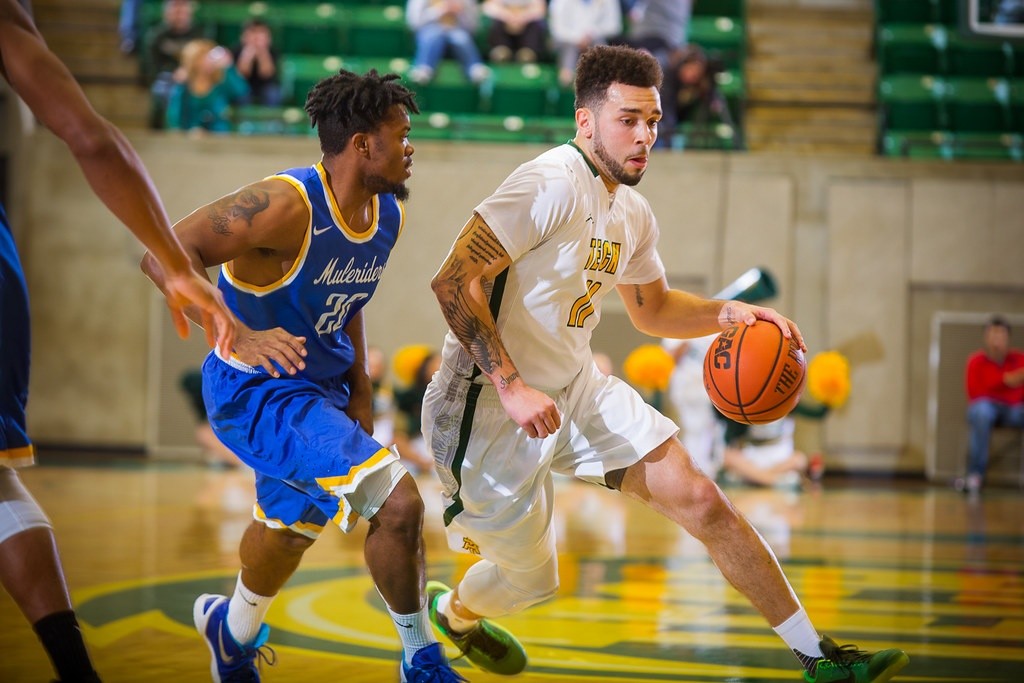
[424, 580, 526, 676]
[399, 642, 469, 683]
[194, 593, 274, 683]
[804, 633, 907, 683]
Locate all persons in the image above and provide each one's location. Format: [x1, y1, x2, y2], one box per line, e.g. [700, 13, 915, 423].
[421, 44, 911, 683]
[965, 314, 1024, 506]
[141, 69, 469, 683]
[121, 0, 731, 149]
[178, 331, 851, 491]
[0, 0, 237, 683]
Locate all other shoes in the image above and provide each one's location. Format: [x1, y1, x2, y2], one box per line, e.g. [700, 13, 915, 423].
[961, 478, 982, 495]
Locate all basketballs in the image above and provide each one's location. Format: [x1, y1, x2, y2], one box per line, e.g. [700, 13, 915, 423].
[701, 319, 809, 427]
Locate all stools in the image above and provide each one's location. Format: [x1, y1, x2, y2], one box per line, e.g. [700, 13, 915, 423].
[970, 421, 1024, 482]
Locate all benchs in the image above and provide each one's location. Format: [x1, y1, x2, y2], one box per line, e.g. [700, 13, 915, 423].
[873, 0, 1023, 162]
[138, 0, 751, 148]
